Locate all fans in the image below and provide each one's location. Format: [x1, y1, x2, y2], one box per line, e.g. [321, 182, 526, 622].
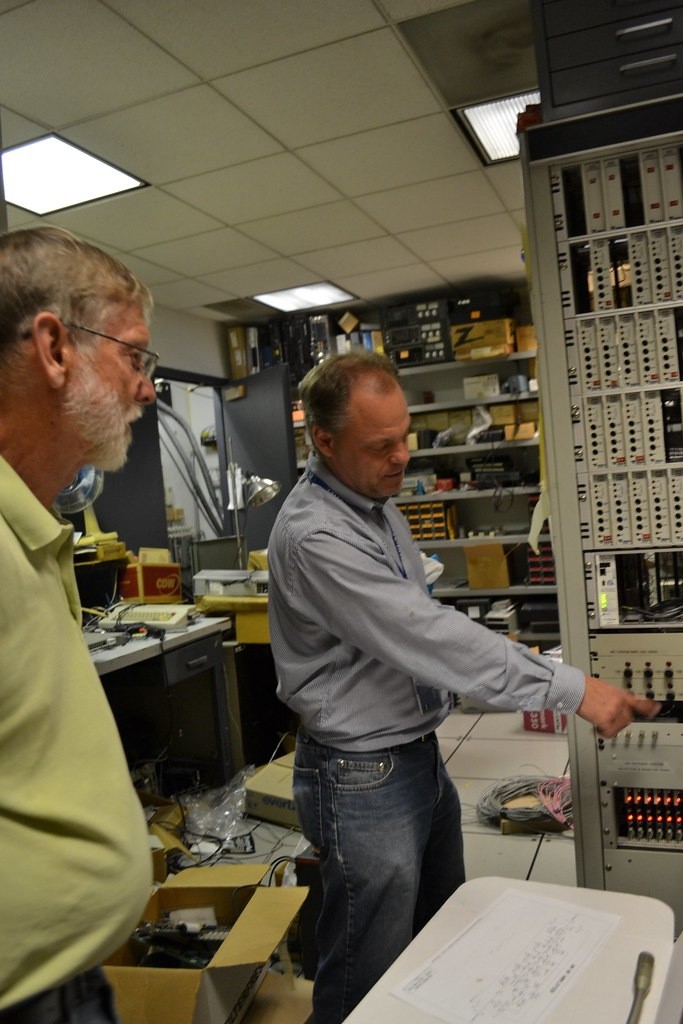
[54, 463, 119, 552]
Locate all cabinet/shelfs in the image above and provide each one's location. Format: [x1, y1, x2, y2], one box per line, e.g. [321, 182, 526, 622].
[291, 347, 566, 643]
[89, 616, 248, 804]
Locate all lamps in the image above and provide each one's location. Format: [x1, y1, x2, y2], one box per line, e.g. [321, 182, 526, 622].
[226, 461, 282, 568]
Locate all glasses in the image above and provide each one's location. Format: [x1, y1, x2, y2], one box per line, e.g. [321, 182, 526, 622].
[19, 318, 161, 383]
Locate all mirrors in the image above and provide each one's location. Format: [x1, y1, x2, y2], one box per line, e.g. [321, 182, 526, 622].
[153, 376, 245, 545]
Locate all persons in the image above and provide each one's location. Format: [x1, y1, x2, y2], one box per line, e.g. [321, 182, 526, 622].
[1, 223, 156, 1024]
[266, 351, 664, 1024]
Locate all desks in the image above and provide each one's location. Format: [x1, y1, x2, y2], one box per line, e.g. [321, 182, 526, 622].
[338, 876, 674, 1024]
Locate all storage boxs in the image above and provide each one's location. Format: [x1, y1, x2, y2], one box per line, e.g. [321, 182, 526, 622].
[238, 749, 300, 829]
[97, 861, 310, 1024]
[118, 562, 183, 604]
